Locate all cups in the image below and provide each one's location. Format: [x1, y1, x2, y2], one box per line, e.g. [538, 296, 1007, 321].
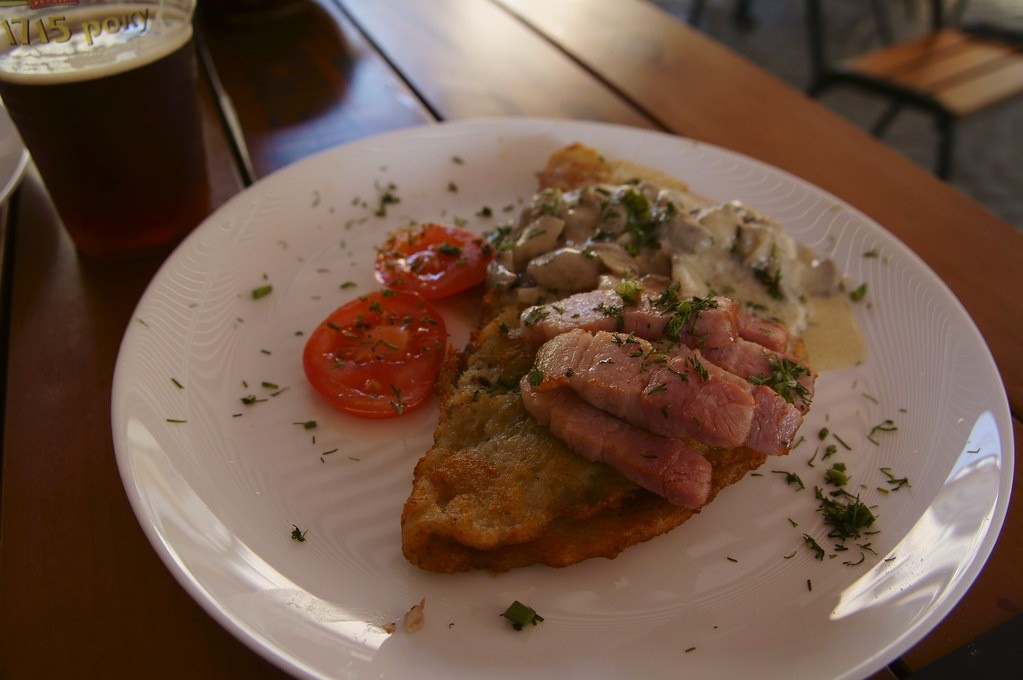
[0, 0, 216, 264]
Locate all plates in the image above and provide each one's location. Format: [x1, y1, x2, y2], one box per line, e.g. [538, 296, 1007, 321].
[0, 95, 31, 208]
[111, 115, 1014, 679]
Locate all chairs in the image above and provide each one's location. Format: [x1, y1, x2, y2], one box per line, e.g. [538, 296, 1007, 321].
[801, 0, 1023, 179]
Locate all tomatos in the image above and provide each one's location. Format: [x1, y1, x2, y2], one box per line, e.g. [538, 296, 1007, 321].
[370, 221, 496, 299]
[302, 287, 447, 416]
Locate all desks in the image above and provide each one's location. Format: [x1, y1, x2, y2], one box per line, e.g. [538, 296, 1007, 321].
[2, 0, 1023, 679]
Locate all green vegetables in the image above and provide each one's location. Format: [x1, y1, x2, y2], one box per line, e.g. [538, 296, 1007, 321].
[228, 154, 918, 631]
